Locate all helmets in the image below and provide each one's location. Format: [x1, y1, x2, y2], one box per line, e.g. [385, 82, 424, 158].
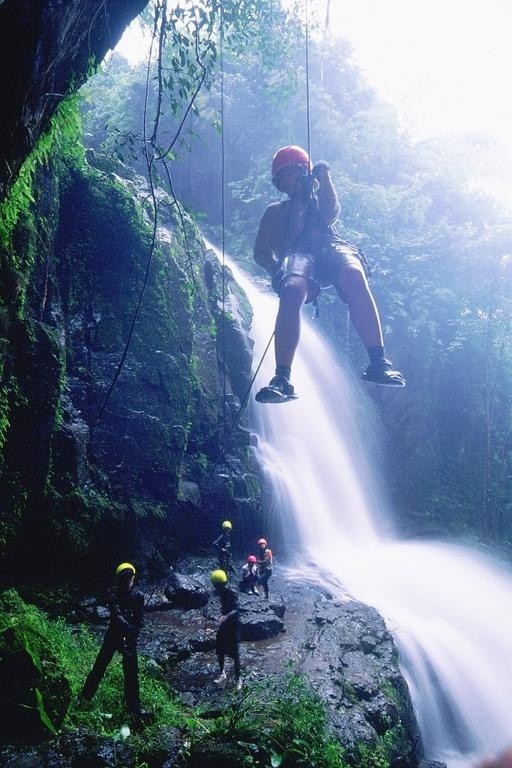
[223, 521, 231, 528]
[211, 570, 227, 584]
[258, 538, 267, 544]
[271, 145, 313, 180]
[116, 563, 135, 576]
[247, 555, 256, 562]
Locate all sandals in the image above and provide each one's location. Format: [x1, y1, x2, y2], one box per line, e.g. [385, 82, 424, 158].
[361, 358, 406, 388]
[255, 375, 299, 403]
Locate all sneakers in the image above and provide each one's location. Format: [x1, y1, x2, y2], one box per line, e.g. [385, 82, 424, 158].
[214, 674, 243, 690]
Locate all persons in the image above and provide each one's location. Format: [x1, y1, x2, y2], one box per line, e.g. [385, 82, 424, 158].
[249, 144, 407, 404]
[78, 561, 145, 715]
[209, 568, 243, 691]
[255, 537, 274, 600]
[240, 555, 261, 595]
[213, 519, 232, 572]
